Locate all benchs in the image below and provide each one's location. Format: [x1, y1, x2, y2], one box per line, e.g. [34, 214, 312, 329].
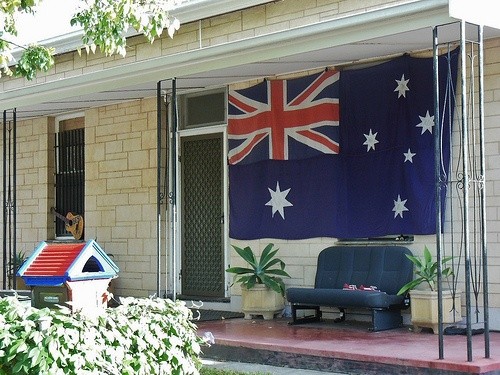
[286, 246, 414, 332]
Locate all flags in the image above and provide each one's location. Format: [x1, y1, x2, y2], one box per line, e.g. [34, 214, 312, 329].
[226, 45, 462, 241]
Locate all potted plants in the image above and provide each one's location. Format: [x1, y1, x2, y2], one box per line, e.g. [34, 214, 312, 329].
[397, 244, 463, 335]
[225, 242, 291, 320]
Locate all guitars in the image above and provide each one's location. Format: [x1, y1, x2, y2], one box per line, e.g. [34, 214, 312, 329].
[50, 207, 83, 239]
